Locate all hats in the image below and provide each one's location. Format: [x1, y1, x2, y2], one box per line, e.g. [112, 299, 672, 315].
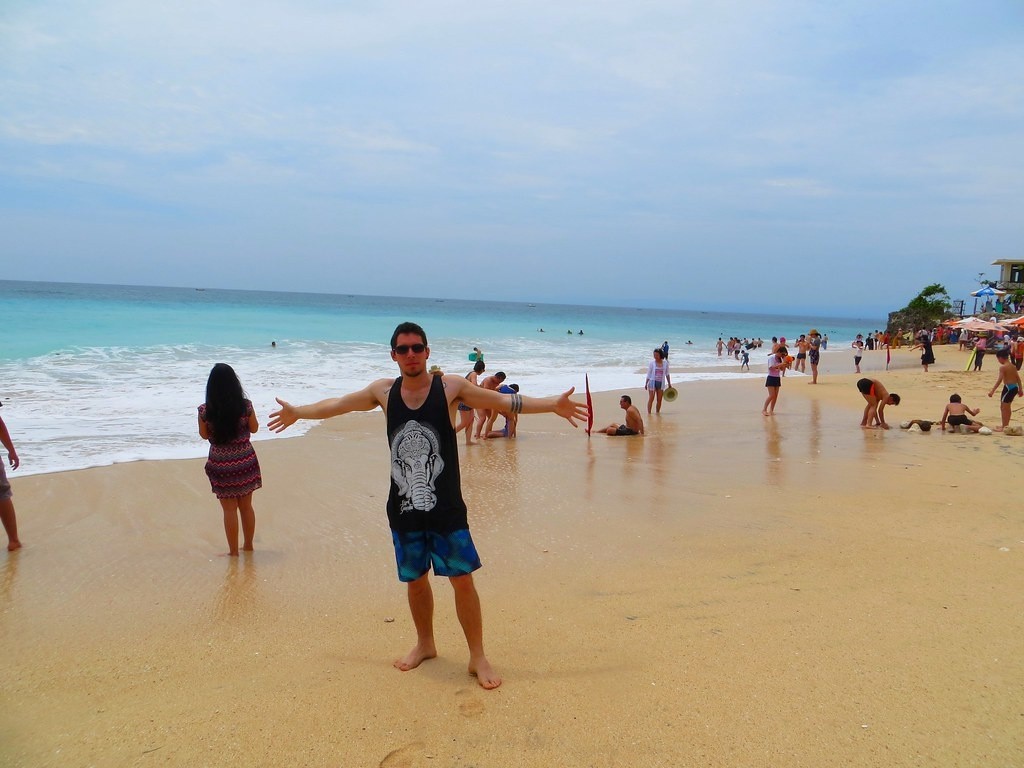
[1017, 337, 1024, 342]
[663, 387, 679, 402]
[808, 329, 818, 335]
[978, 331, 988, 337]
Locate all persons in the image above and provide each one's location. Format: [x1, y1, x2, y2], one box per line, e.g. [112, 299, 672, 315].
[475, 372, 506, 440]
[474, 419, 516, 439]
[578, 329, 584, 335]
[980, 296, 1024, 315]
[584, 395, 644, 436]
[197, 363, 262, 557]
[539, 329, 544, 332]
[662, 341, 669, 361]
[483, 384, 519, 440]
[267, 321, 591, 690]
[568, 329, 572, 334]
[0, 401, 21, 552]
[456, 360, 486, 446]
[645, 348, 672, 414]
[474, 347, 481, 362]
[685, 324, 1024, 435]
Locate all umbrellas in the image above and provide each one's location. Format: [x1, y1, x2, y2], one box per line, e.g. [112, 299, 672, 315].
[971, 286, 1006, 297]
[942, 315, 1024, 337]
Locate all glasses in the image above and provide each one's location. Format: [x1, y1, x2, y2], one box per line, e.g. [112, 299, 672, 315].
[393, 344, 426, 354]
